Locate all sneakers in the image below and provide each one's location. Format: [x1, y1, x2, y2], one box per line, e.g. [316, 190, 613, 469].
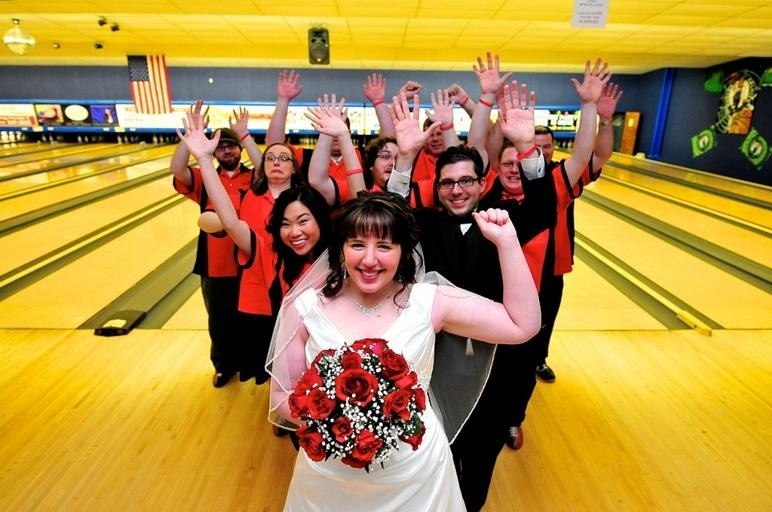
[213, 371, 237, 387]
[272, 424, 289, 436]
[537, 365, 556, 381]
[506, 427, 524, 449]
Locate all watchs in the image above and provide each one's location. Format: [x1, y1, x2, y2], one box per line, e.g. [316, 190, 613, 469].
[599, 121, 614, 126]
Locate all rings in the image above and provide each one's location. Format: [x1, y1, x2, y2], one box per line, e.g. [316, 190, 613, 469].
[609, 97, 613, 99]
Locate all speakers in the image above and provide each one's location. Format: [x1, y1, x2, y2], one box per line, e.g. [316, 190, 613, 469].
[308, 28, 329, 65]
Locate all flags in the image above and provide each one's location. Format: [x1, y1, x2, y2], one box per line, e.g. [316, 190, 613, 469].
[128, 54, 172, 115]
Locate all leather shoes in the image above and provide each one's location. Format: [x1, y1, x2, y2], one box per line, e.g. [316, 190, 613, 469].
[239, 372, 254, 382]
[255, 375, 268, 385]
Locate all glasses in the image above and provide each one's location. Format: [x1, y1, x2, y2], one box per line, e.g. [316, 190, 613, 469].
[375, 154, 398, 161]
[263, 155, 295, 163]
[436, 178, 482, 189]
[216, 143, 239, 151]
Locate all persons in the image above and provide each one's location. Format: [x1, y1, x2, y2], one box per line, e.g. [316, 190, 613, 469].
[487, 82, 623, 382]
[194, 178, 267, 246]
[169, 99, 261, 387]
[305, 90, 463, 221]
[268, 192, 542, 512]
[368, 79, 556, 502]
[469, 50, 612, 450]
[388, 77, 497, 184]
[261, 71, 395, 200]
[180, 114, 337, 315]
[234, 143, 300, 384]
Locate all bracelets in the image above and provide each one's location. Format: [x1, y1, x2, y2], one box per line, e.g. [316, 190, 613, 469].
[476, 99, 494, 106]
[237, 132, 249, 140]
[515, 144, 536, 162]
[369, 99, 385, 106]
[345, 169, 366, 178]
[438, 123, 455, 130]
[457, 97, 468, 106]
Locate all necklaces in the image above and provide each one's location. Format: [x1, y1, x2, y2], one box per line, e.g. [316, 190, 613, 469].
[345, 292, 397, 318]
